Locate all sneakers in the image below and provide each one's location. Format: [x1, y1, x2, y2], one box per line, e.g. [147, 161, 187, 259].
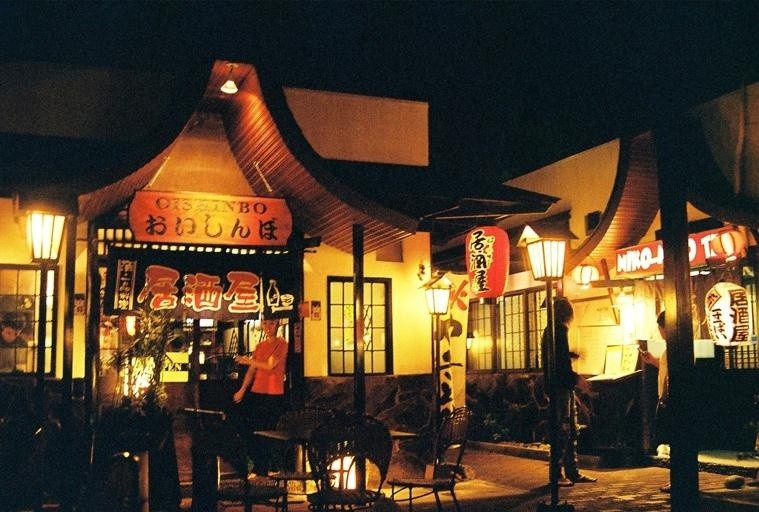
[661, 485, 670, 492]
[557, 475, 597, 485]
[247, 471, 281, 479]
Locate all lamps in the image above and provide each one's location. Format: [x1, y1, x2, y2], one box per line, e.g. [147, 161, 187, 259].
[220, 63, 239, 95]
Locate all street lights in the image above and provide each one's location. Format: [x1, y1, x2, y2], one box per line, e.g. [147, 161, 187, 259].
[526, 236, 574, 511]
[425, 276, 453, 465]
[25, 210, 66, 391]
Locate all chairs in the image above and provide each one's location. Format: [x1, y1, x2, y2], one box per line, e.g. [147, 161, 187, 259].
[212, 404, 471, 512]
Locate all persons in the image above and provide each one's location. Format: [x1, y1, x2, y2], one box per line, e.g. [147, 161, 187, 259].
[0, 311, 28, 370]
[638, 309, 673, 493]
[539, 300, 598, 487]
[231, 311, 291, 479]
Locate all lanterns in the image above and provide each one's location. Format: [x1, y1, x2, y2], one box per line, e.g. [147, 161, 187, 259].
[711, 228, 745, 265]
[703, 278, 755, 351]
[465, 218, 511, 305]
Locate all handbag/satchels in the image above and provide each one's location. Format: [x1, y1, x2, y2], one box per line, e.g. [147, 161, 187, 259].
[648, 416, 670, 450]
